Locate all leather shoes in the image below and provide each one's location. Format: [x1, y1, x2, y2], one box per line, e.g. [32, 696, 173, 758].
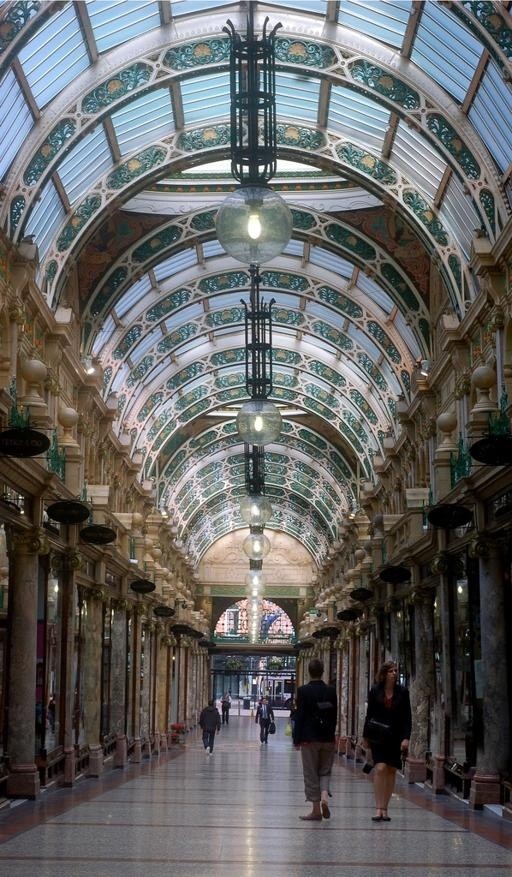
[260, 738, 268, 744]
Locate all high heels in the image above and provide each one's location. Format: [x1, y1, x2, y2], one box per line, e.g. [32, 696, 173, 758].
[371, 808, 391, 821]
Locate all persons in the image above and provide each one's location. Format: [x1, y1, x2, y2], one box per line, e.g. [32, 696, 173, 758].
[220, 692, 232, 724]
[200, 700, 221, 756]
[362, 661, 412, 821]
[255, 698, 274, 744]
[292, 658, 337, 821]
[289, 698, 297, 737]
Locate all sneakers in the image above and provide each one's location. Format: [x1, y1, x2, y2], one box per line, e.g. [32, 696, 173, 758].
[299, 800, 331, 820]
[204, 746, 213, 760]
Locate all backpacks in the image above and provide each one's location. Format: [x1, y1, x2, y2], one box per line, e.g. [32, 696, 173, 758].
[305, 683, 334, 733]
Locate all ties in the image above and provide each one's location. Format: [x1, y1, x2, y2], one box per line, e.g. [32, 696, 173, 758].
[263, 704, 266, 720]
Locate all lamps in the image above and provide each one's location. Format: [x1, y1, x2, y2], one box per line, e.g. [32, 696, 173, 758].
[242, 443, 270, 590]
[238, 298, 282, 450]
[213, 18, 294, 268]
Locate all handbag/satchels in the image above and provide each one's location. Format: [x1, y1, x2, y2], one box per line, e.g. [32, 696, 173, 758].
[361, 717, 391, 747]
[268, 721, 276, 734]
